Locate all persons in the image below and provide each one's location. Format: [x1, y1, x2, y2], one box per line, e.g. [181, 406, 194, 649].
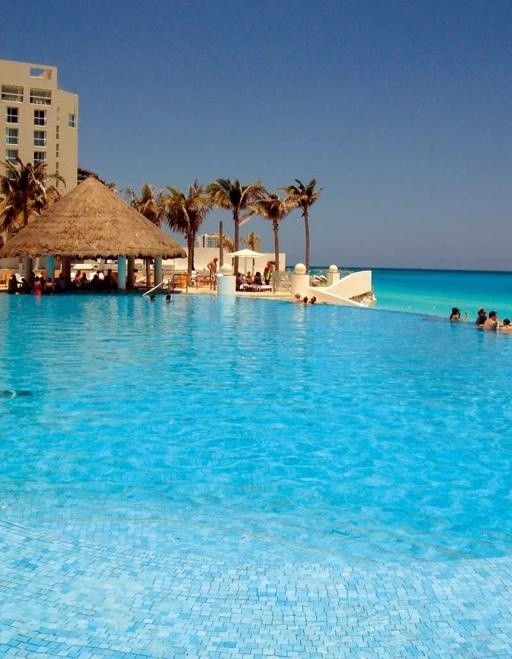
[8, 269, 138, 295]
[309, 296, 316, 304]
[195, 257, 275, 291]
[450, 307, 512, 332]
[303, 296, 308, 304]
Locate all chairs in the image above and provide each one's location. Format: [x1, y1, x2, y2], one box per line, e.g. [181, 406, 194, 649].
[55, 270, 62, 279]
[134, 267, 328, 292]
[0, 268, 14, 288]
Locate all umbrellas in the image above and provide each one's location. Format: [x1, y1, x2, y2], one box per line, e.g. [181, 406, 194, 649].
[225, 249, 264, 274]
